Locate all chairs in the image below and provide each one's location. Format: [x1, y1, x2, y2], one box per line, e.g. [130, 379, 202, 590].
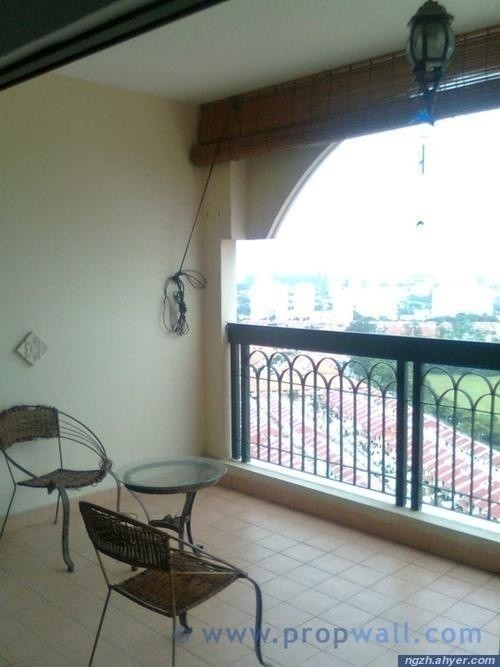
[77, 500, 273, 667]
[1, 404, 121, 572]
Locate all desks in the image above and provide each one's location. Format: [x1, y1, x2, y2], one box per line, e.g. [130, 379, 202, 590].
[112, 458, 228, 558]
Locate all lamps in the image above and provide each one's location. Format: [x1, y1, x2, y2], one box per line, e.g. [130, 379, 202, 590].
[406, 0, 455, 116]
